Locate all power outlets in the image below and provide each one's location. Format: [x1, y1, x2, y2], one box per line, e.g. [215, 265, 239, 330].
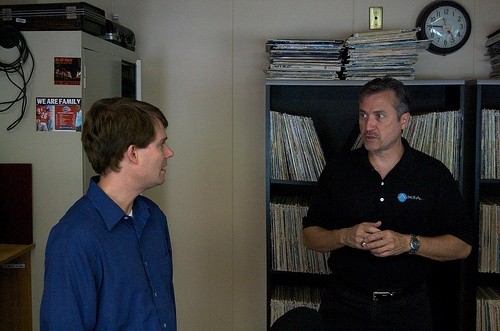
[369, 8, 383, 29]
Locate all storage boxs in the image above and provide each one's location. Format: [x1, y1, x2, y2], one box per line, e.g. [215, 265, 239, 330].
[104, 19, 136, 52]
[0, 2, 106, 37]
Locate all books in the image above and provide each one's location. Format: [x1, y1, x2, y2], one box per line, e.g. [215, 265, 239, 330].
[476, 285, 500, 331]
[271, 280, 323, 327]
[269, 195, 332, 275]
[269, 111, 325, 182]
[484, 28, 500, 80]
[265, 27, 433, 80]
[401, 109, 461, 181]
[478, 201, 500, 273]
[338, 132, 364, 151]
[481, 109, 500, 180]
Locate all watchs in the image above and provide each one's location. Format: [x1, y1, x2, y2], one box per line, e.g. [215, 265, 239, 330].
[410, 233, 421, 256]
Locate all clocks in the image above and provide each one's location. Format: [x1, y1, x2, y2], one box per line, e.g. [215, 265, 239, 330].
[419, 1, 472, 56]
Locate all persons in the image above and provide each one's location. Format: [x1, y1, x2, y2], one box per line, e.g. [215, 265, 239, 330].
[75, 104, 82, 132]
[303, 78, 474, 331]
[39, 96, 176, 331]
[36, 104, 54, 132]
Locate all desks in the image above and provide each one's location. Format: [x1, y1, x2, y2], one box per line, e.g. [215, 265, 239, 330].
[0, 244, 35, 331]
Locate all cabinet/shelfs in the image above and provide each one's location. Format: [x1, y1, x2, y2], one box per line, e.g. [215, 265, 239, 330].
[0, 30, 142, 331]
[264, 79, 500, 331]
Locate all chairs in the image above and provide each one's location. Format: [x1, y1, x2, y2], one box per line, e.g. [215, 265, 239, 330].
[268, 307, 318, 331]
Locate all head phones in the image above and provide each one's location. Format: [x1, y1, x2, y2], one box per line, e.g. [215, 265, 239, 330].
[0, 23, 28, 73]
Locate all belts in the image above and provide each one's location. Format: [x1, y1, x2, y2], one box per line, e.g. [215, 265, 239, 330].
[330, 275, 424, 303]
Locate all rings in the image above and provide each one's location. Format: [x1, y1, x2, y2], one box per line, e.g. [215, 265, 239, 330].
[360, 241, 367, 247]
